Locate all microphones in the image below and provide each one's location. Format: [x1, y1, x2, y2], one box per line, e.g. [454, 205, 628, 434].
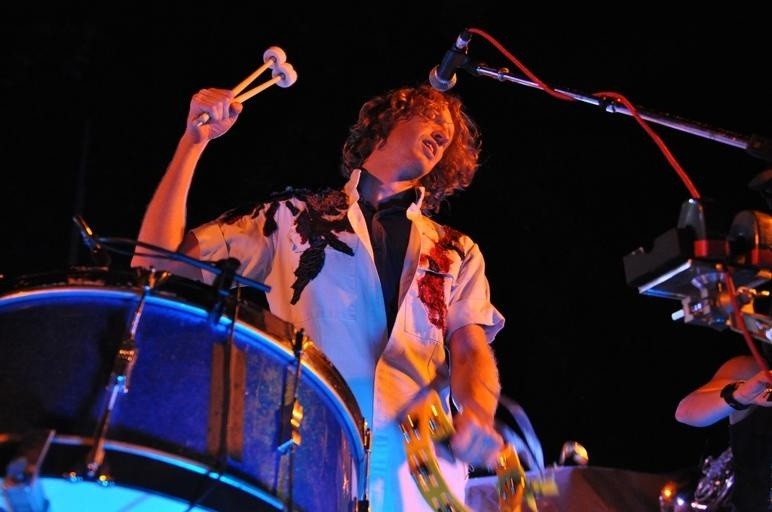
[428, 29, 472, 91]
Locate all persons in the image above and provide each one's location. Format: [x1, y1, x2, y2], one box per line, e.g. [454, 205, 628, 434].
[673, 353, 771, 512]
[129, 82, 506, 511]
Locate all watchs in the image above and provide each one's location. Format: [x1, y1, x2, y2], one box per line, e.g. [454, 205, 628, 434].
[720, 382, 751, 410]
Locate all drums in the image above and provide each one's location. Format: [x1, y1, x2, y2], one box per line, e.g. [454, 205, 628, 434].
[1, 266, 371, 512]
